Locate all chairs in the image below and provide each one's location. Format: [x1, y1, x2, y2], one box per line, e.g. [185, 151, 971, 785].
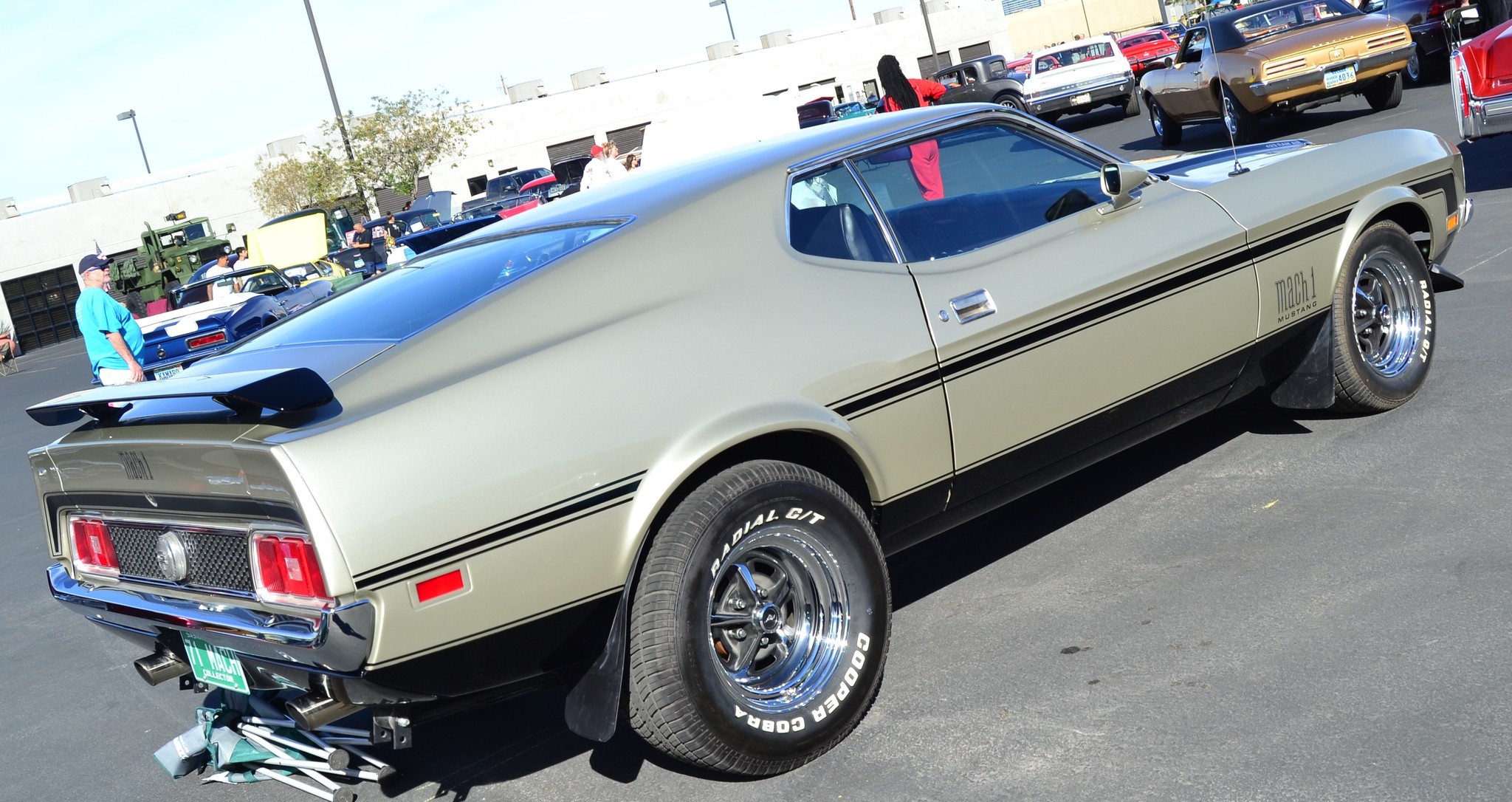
[789, 202, 895, 265]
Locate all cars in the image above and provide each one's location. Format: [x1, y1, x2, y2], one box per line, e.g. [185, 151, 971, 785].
[796, 0, 1246, 163]
[180, 252, 240, 308]
[343, 186, 457, 276]
[1444, 0, 1511, 141]
[236, 212, 352, 295]
[497, 173, 559, 220]
[1358, 0, 1462, 84]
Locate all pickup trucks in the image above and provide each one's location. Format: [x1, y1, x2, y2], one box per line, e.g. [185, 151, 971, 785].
[461, 167, 554, 220]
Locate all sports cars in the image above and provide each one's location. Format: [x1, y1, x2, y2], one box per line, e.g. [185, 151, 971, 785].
[92, 262, 337, 385]
[1140, 0, 1416, 140]
[27, 98, 1476, 786]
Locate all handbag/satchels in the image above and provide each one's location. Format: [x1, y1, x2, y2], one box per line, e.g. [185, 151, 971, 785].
[385, 227, 395, 247]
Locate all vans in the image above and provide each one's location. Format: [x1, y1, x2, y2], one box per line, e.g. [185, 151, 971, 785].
[256, 203, 357, 255]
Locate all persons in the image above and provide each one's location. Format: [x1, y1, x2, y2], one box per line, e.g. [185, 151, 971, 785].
[877, 54, 947, 201]
[867, 90, 877, 103]
[1024, 49, 1034, 59]
[406, 201, 412, 208]
[233, 246, 265, 292]
[580, 145, 611, 192]
[0, 342, 11, 362]
[626, 153, 638, 172]
[349, 222, 381, 277]
[602, 139, 628, 177]
[1180, 0, 1323, 42]
[949, 73, 970, 88]
[206, 251, 240, 301]
[402, 206, 409, 211]
[384, 211, 401, 241]
[1044, 30, 1117, 65]
[74, 254, 148, 386]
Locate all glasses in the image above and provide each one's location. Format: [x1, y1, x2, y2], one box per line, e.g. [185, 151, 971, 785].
[222, 258, 229, 261]
[86, 264, 110, 271]
[633, 158, 636, 161]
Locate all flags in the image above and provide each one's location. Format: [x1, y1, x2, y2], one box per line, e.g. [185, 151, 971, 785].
[96, 243, 111, 292]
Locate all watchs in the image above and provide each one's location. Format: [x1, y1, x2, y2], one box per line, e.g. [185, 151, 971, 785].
[260, 281, 264, 285]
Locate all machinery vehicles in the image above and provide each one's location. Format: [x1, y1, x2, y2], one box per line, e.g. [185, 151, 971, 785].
[109, 211, 236, 318]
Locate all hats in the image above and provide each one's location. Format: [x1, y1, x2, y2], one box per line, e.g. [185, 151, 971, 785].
[78, 254, 114, 275]
[590, 145, 603, 156]
